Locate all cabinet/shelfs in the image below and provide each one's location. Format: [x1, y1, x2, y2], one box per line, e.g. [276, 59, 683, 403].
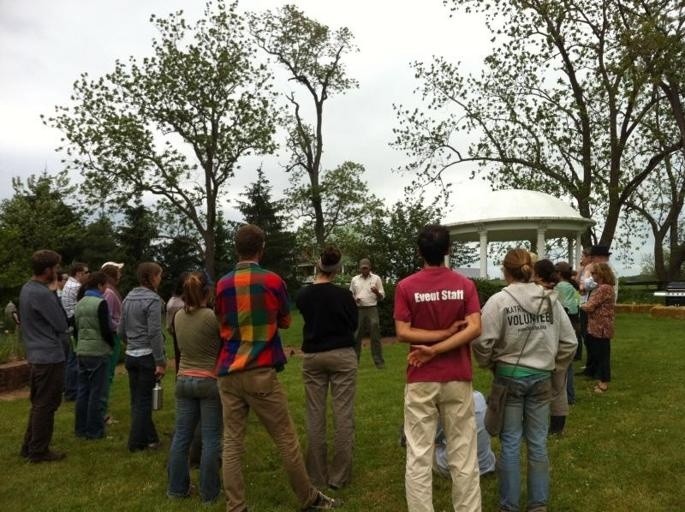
[151, 379, 164, 411]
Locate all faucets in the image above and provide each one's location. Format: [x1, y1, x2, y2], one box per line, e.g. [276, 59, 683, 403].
[594, 384, 608, 394]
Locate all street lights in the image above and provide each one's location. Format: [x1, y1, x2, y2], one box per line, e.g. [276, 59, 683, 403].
[484, 382, 508, 436]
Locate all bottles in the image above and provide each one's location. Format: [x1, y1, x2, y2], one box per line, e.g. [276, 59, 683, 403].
[201, 271, 215, 289]
[101, 262, 125, 269]
[555, 262, 571, 272]
[587, 246, 612, 256]
[360, 258, 370, 270]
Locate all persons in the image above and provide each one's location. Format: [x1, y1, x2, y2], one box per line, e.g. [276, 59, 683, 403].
[347, 258, 387, 370]
[392, 223, 483, 512]
[117, 262, 167, 453]
[166, 271, 185, 381]
[429, 387, 496, 479]
[62, 262, 91, 402]
[167, 271, 219, 502]
[47, 272, 58, 291]
[56, 269, 69, 301]
[73, 271, 116, 441]
[102, 261, 124, 424]
[210, 224, 347, 512]
[529, 243, 619, 434]
[208, 285, 216, 309]
[20, 250, 69, 463]
[473, 248, 578, 510]
[295, 241, 358, 492]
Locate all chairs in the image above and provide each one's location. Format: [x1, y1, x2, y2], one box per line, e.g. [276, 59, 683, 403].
[301, 491, 345, 512]
[32, 452, 66, 463]
